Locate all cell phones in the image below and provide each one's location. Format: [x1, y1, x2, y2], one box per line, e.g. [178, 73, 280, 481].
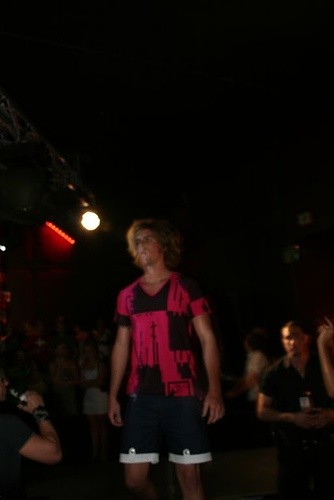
[16, 393, 28, 405]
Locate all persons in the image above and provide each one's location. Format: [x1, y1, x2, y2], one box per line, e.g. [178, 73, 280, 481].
[80, 340, 109, 462]
[226, 328, 271, 405]
[69, 316, 113, 359]
[0, 369, 62, 500]
[317, 316, 334, 398]
[257, 319, 334, 500]
[106, 219, 224, 500]
[0, 286, 12, 362]
[50, 340, 76, 417]
